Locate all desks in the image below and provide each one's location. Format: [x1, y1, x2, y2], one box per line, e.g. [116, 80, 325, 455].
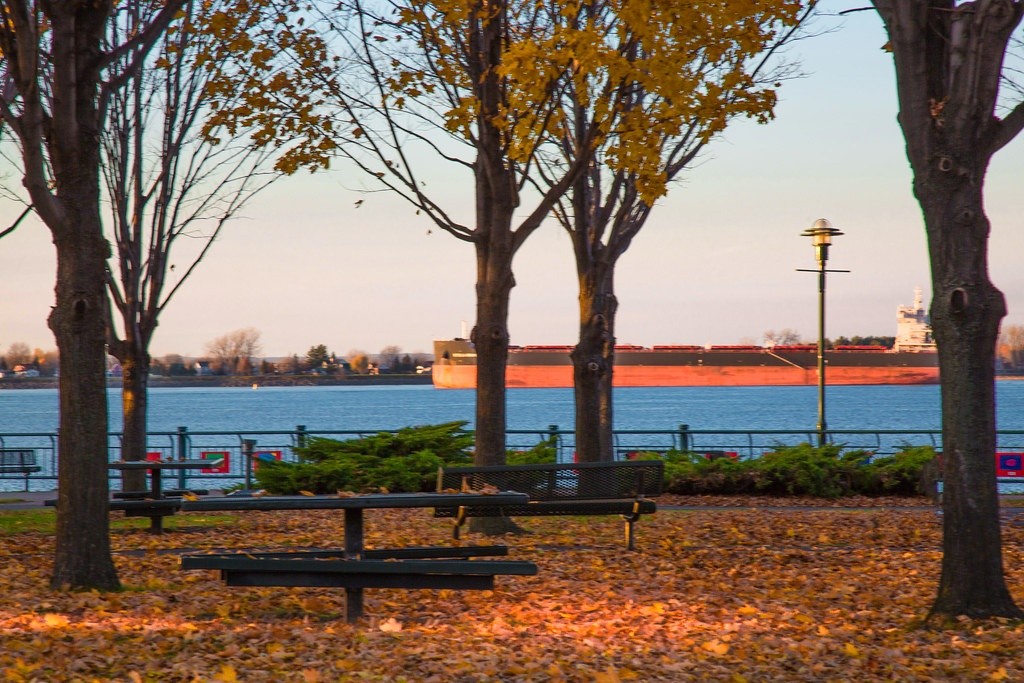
[183, 493, 529, 625]
[107, 458, 226, 535]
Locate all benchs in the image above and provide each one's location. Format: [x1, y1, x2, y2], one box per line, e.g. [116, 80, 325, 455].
[228, 546, 508, 563]
[0, 448, 41, 491]
[43, 499, 184, 517]
[113, 488, 210, 498]
[182, 555, 537, 616]
[435, 459, 665, 549]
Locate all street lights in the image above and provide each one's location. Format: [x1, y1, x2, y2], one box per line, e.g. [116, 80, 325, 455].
[794, 218, 853, 447]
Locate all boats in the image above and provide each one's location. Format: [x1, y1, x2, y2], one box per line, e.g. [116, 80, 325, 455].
[430, 338, 940, 389]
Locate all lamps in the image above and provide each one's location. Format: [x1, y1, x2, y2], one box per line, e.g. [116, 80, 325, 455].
[936, 478, 944, 493]
